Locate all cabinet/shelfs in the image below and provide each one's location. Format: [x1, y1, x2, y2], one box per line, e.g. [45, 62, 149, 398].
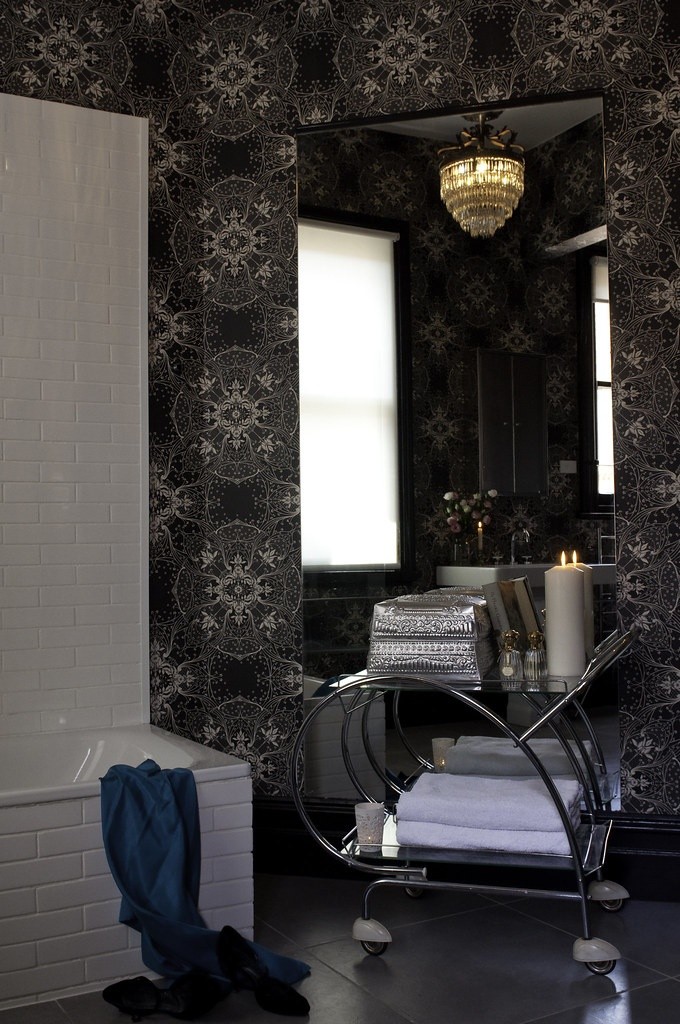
[289, 665, 630, 975]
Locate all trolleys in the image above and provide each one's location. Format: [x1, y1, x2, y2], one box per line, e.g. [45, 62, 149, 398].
[287, 609, 643, 976]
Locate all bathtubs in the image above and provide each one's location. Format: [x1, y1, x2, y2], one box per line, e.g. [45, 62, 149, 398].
[3, 718, 255, 1017]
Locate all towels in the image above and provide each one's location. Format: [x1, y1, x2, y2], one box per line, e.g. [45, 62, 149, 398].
[101, 759, 310, 1024]
[395, 770, 583, 859]
[446, 738, 593, 777]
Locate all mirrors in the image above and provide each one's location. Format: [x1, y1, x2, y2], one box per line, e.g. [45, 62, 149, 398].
[294, 88, 623, 813]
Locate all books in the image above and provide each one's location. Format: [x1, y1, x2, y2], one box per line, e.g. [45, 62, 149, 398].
[511, 574, 547, 649]
[482, 578, 528, 655]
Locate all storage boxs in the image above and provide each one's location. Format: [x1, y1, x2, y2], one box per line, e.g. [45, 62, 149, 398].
[482, 573, 542, 659]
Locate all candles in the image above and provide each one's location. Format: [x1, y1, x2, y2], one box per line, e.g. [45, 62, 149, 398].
[567, 550, 595, 661]
[477, 520, 484, 549]
[543, 554, 585, 675]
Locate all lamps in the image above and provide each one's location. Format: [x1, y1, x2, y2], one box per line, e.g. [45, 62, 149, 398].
[437, 110, 526, 241]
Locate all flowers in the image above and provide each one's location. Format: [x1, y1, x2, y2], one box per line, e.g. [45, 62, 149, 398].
[443, 489, 498, 533]
